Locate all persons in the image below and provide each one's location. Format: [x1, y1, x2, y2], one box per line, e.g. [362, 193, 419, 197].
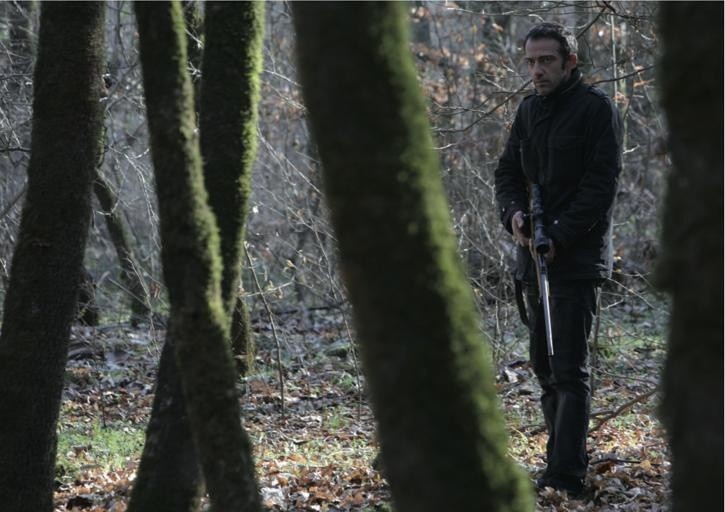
[494, 21, 624, 499]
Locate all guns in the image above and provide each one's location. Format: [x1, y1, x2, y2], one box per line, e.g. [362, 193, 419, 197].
[526, 184, 555, 355]
